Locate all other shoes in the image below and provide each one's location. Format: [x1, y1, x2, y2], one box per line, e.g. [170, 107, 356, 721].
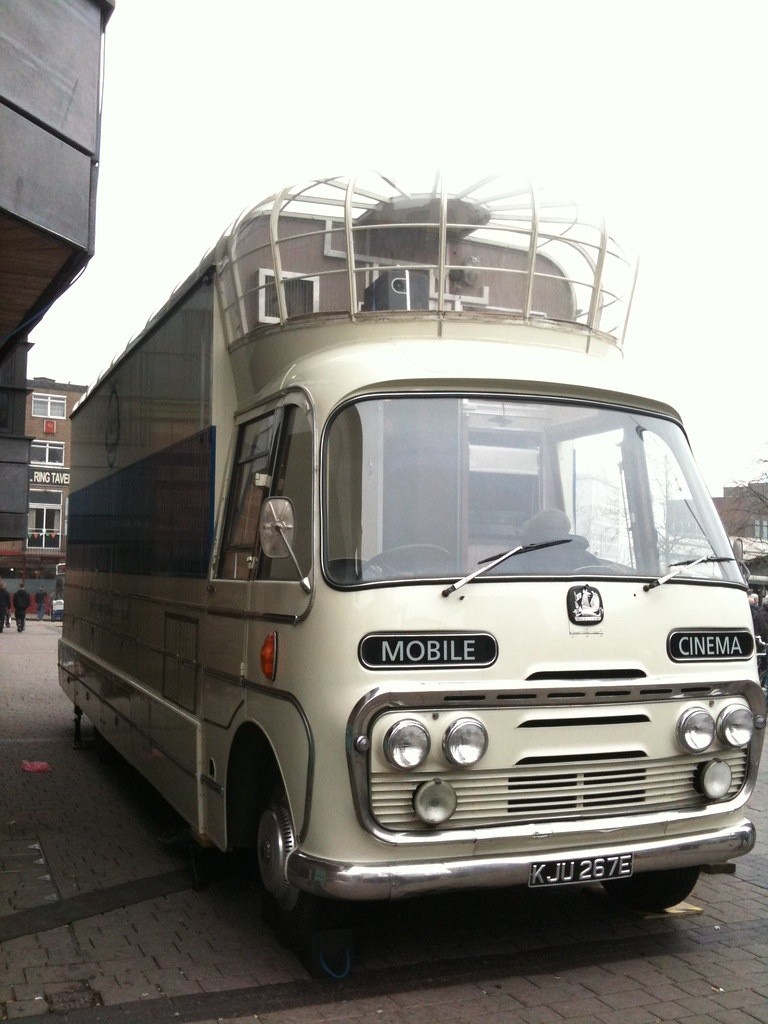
[17, 624, 21, 630]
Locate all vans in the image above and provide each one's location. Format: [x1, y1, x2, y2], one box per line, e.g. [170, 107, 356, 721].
[61, 171, 766, 932]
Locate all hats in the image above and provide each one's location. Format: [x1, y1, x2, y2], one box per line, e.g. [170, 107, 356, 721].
[518, 507, 590, 551]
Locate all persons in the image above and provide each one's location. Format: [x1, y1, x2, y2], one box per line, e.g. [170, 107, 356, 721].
[0, 580, 11, 633]
[35, 585, 47, 621]
[13, 584, 30, 632]
[55, 576, 64, 599]
[749, 594, 768, 686]
